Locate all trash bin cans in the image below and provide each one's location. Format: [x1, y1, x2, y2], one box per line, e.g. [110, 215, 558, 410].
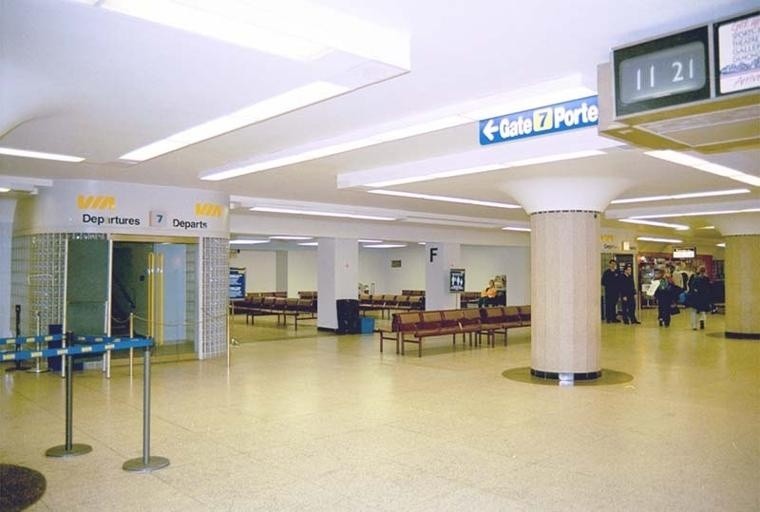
[360, 317, 375, 334]
[336, 299, 361, 335]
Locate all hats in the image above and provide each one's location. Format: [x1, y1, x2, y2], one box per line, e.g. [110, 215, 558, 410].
[625, 262, 632, 268]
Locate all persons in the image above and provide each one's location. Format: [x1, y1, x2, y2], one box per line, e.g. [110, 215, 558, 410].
[601, 259, 641, 324]
[653, 277, 677, 328]
[688, 266, 719, 331]
[478, 275, 506, 307]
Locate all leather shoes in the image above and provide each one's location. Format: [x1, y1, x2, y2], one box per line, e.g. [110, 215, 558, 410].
[607, 319, 641, 324]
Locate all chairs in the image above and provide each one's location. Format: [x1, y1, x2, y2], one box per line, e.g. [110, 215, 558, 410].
[228, 291, 317, 330]
[373, 303, 531, 356]
[461, 291, 481, 309]
[358, 290, 425, 321]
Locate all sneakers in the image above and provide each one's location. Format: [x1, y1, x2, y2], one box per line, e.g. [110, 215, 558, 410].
[699, 317, 705, 329]
